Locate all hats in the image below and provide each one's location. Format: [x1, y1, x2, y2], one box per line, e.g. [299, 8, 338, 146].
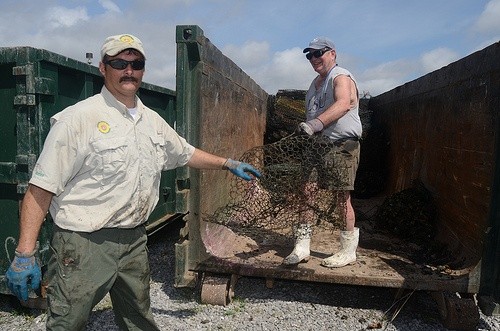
[303, 36, 336, 53]
[100, 34, 146, 62]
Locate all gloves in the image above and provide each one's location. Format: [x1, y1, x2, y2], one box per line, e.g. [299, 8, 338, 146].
[295, 117, 325, 139]
[222, 157, 261, 181]
[4, 249, 42, 305]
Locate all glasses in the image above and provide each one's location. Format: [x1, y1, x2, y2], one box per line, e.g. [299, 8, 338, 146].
[104, 59, 145, 71]
[306, 49, 330, 60]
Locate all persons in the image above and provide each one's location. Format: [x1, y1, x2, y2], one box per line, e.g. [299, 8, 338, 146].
[5, 33, 262, 331]
[282, 36, 362, 268]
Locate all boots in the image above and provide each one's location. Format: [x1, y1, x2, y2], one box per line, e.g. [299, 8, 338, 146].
[321, 227, 360, 268]
[283, 224, 312, 264]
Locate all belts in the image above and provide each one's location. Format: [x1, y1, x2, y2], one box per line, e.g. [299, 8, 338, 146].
[342, 137, 358, 142]
